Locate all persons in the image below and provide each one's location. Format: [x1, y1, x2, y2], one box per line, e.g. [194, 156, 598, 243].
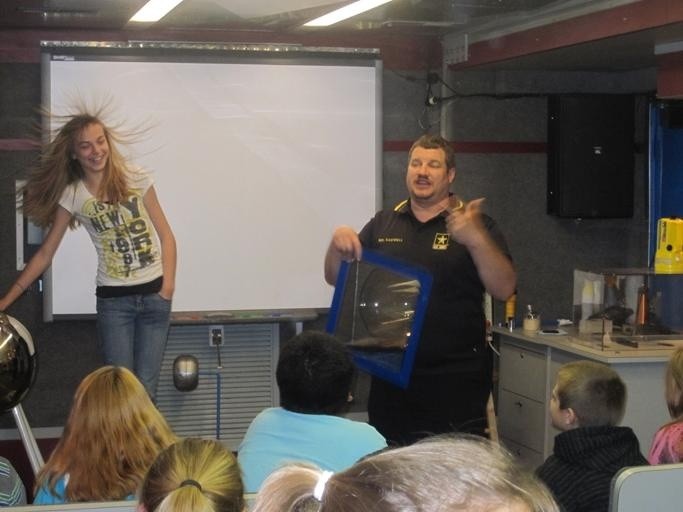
[252, 431, 565, 512]
[324, 135, 517, 447]
[236, 330, 388, 493]
[647, 346, 682, 464]
[30, 364, 179, 506]
[138, 438, 244, 512]
[0, 455, 28, 508]
[1, 83, 177, 404]
[533, 359, 650, 512]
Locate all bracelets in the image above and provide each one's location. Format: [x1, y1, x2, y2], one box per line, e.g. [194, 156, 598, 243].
[14, 282, 25, 294]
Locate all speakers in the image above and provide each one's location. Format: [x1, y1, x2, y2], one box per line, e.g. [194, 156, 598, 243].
[547, 95, 635, 219]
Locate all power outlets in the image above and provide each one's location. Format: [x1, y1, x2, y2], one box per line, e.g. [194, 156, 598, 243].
[209, 325, 224, 347]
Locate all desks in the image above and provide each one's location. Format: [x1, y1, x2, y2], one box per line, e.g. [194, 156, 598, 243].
[491, 324, 683, 478]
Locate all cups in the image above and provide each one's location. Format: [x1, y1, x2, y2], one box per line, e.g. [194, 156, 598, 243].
[522, 313, 543, 331]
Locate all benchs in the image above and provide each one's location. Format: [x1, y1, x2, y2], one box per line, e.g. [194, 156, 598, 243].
[0, 493, 255, 512]
[609, 463, 683, 512]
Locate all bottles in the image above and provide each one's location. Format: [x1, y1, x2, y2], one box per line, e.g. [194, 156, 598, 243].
[581, 275, 649, 328]
[504, 288, 518, 330]
